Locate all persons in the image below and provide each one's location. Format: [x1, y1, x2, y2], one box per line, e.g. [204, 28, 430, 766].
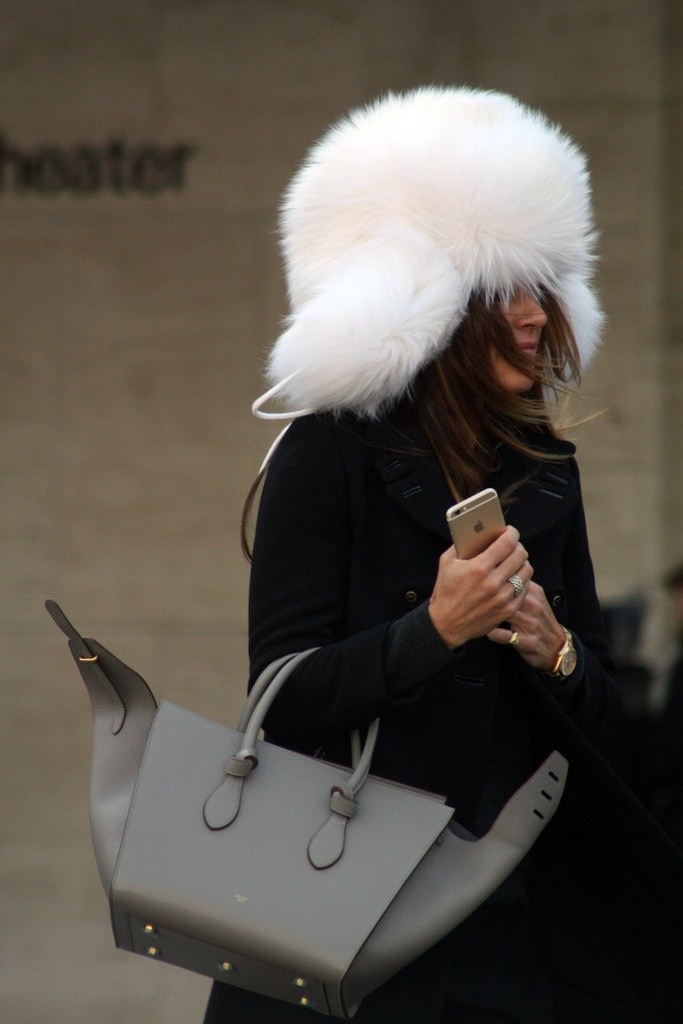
[198, 85, 620, 1024]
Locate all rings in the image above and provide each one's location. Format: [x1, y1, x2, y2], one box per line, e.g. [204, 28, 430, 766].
[507, 574, 525, 596]
[509, 631, 520, 647]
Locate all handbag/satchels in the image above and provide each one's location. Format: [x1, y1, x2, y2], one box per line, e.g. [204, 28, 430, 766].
[45, 599, 570, 1020]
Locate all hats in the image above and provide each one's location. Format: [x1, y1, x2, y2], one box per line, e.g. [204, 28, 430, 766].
[240, 84, 602, 471]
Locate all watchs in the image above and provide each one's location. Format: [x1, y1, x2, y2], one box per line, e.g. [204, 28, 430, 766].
[535, 625, 577, 683]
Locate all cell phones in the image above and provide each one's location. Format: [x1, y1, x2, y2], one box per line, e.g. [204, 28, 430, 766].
[446, 488, 506, 561]
